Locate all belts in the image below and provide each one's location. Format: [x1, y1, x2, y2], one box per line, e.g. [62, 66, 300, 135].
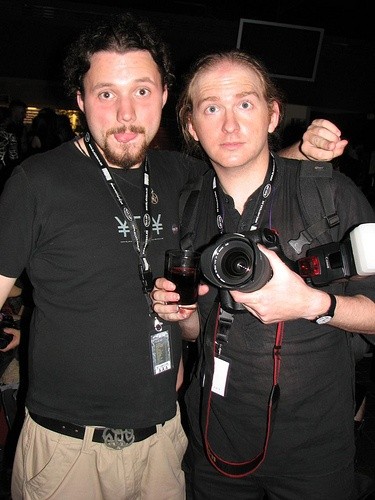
[27, 409, 166, 451]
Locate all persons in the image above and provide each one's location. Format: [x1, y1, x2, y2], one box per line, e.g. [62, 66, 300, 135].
[0, 12, 375, 500]
[151, 47, 375, 500]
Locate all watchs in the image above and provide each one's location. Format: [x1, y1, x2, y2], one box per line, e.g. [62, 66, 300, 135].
[309, 292, 336, 325]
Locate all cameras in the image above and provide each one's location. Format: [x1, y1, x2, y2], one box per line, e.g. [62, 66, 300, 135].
[199, 221, 375, 314]
